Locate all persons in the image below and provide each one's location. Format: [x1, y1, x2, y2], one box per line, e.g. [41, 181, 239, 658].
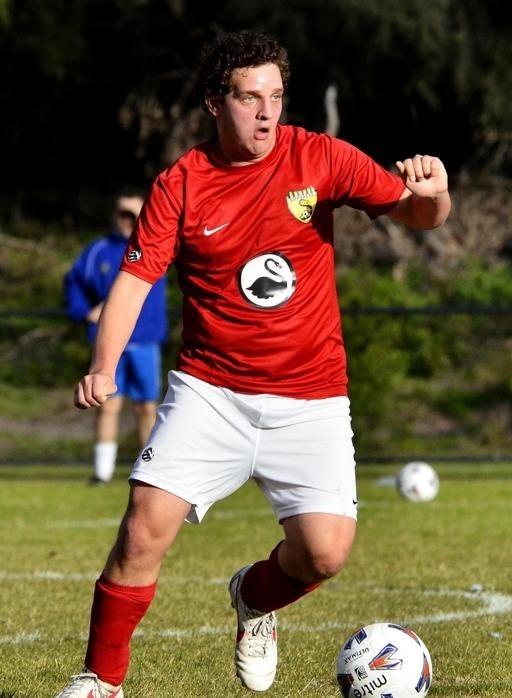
[53, 31, 452, 698]
[62, 195, 170, 487]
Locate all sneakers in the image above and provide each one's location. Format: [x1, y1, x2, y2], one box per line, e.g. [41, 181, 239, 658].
[229, 565, 279, 693]
[50, 672, 128, 698]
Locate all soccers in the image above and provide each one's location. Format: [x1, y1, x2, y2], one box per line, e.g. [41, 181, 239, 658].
[337, 623, 433, 698]
[396, 461, 439, 504]
